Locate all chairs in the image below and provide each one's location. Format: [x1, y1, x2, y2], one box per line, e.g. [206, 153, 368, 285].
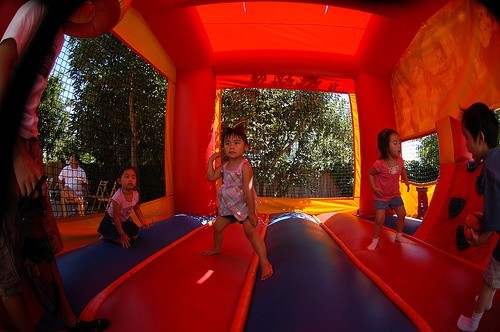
[88, 180, 109, 211]
[48, 189, 60, 216]
[97, 181, 117, 210]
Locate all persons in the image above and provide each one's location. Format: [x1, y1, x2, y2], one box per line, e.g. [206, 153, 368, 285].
[367, 128, 415, 250]
[458, 102, 500, 332]
[203, 128, 273, 280]
[1, 0, 111, 332]
[97, 167, 150, 248]
[57, 154, 89, 217]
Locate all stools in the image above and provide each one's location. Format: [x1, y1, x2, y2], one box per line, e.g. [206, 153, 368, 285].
[66, 203, 78, 216]
